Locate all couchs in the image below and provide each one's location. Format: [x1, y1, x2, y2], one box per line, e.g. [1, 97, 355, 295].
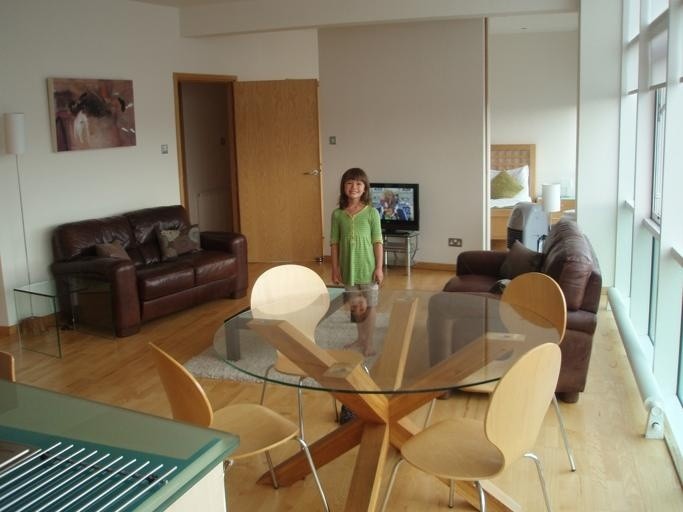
[49, 205, 249, 338]
[425, 219, 605, 406]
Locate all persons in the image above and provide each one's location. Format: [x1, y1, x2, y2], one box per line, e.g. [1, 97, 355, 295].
[342, 289, 370, 322]
[375, 191, 410, 219]
[330, 167, 384, 357]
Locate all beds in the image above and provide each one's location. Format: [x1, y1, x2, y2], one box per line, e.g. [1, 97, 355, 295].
[489, 143, 536, 241]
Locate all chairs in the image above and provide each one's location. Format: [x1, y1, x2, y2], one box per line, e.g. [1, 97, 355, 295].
[0, 351, 15, 384]
[423, 273, 578, 475]
[382, 343, 562, 511]
[249, 263, 372, 482]
[148, 341, 332, 511]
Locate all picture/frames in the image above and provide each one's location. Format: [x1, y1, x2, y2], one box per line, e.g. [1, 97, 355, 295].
[45, 75, 137, 154]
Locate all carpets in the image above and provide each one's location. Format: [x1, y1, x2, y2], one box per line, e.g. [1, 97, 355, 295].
[177, 287, 392, 392]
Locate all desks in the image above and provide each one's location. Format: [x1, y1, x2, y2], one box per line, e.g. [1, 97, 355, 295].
[11, 275, 117, 358]
[0, 380, 241, 512]
[210, 285, 564, 512]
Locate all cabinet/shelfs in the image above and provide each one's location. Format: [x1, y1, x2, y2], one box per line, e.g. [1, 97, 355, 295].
[380, 231, 421, 277]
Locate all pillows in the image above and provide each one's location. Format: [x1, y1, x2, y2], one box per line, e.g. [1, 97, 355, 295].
[490, 164, 529, 199]
[490, 169, 523, 200]
[91, 238, 133, 264]
[488, 278, 512, 295]
[495, 236, 546, 282]
[152, 222, 205, 264]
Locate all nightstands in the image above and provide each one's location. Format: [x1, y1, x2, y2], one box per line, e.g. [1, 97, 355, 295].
[538, 198, 576, 227]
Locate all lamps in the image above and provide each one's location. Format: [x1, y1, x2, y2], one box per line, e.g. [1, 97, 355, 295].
[540, 183, 563, 234]
[0, 110, 48, 338]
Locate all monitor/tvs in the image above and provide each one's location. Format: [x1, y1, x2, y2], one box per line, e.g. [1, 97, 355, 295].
[368, 183, 418, 237]
